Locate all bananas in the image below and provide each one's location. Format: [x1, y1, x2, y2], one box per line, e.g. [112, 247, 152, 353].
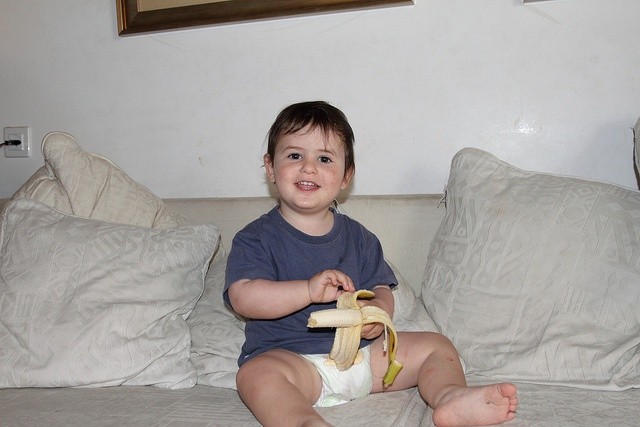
[305, 288, 404, 392]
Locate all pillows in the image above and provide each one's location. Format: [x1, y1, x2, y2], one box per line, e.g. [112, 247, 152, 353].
[414, 145, 639, 395]
[1, 197, 220, 390]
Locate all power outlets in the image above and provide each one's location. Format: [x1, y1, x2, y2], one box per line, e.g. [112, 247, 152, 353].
[4, 125, 33, 158]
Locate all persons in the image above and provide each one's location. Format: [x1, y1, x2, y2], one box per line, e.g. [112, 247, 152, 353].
[222, 100, 518, 427]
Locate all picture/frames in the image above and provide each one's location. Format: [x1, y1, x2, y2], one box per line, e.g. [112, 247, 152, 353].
[116, 0, 420, 39]
